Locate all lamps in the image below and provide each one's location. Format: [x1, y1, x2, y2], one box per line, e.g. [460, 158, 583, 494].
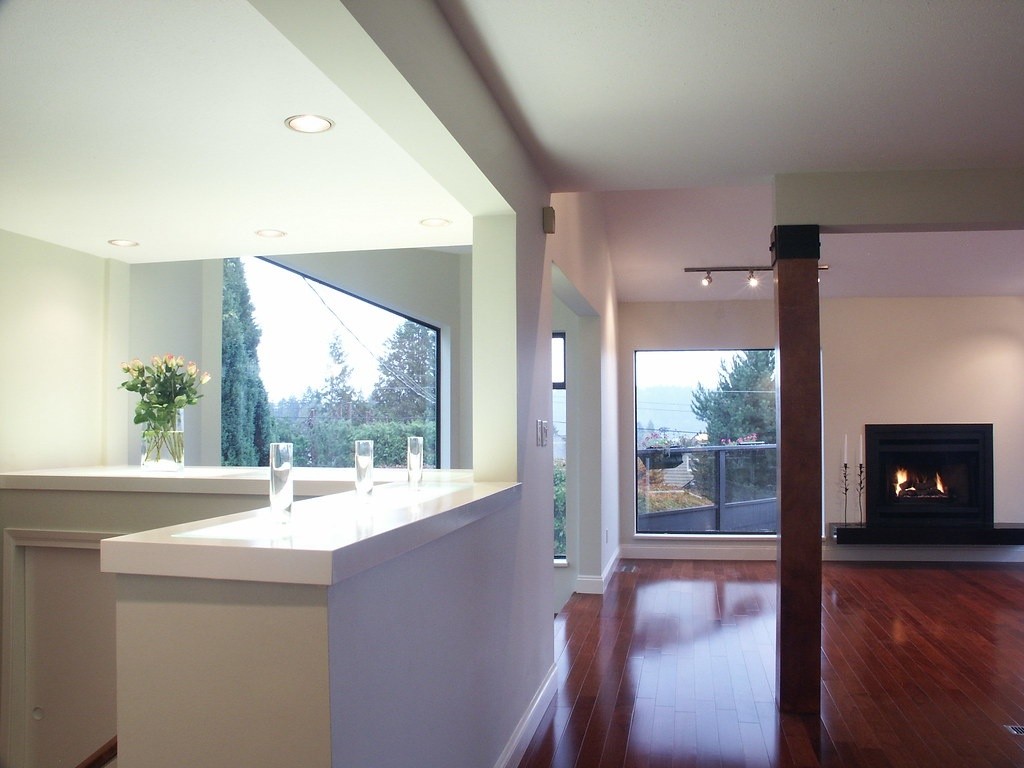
[748, 276, 757, 286]
[702, 276, 712, 285]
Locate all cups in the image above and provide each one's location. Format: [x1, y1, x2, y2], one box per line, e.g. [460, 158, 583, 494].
[269, 442, 293, 510]
[407, 437, 423, 483]
[354, 440, 374, 494]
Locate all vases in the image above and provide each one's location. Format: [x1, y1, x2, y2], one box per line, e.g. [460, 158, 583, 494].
[355, 439, 375, 497]
[141, 431, 185, 472]
[640, 446, 682, 470]
[729, 442, 766, 466]
[407, 437, 424, 491]
[270, 442, 294, 524]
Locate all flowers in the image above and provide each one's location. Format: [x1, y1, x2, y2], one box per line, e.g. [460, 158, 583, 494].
[722, 433, 757, 446]
[642, 433, 673, 446]
[119, 353, 211, 464]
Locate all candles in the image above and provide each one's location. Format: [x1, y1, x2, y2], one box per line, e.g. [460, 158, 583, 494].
[859, 434, 863, 464]
[844, 434, 848, 463]
